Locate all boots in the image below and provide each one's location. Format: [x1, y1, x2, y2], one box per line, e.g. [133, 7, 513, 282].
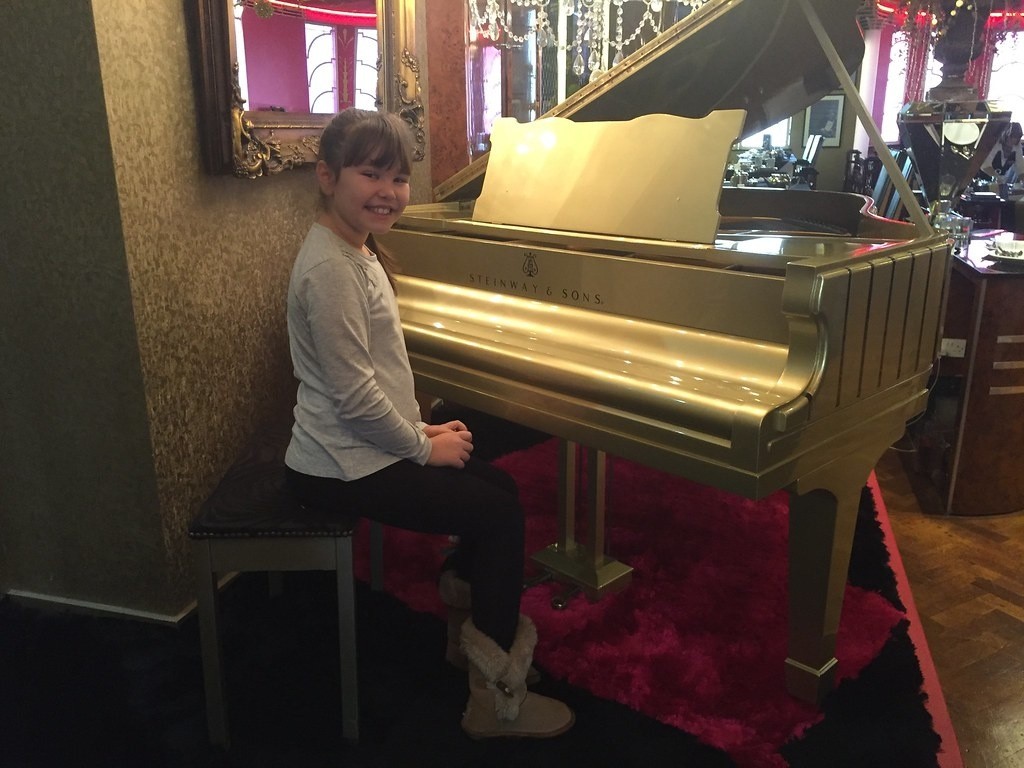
[438, 569, 472, 670]
[460, 612, 575, 738]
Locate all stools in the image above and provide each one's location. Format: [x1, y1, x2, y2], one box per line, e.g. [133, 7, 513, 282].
[189, 424, 385, 752]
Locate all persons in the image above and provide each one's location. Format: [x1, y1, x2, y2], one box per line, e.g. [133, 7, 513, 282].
[284, 107, 573, 739]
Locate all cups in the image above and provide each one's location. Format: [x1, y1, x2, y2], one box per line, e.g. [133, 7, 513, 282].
[941, 217, 971, 238]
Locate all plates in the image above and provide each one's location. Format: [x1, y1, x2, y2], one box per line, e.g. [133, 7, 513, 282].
[988, 241, 1024, 264]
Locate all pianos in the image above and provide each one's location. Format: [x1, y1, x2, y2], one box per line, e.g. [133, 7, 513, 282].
[373, 0, 957, 706]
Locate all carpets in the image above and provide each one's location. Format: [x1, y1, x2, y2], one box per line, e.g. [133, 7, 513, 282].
[353, 438, 904, 768]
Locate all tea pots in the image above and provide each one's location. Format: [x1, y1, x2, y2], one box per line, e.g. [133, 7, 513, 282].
[764, 173, 790, 188]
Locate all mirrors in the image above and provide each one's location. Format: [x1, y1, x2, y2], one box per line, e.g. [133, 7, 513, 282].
[183, 0, 430, 180]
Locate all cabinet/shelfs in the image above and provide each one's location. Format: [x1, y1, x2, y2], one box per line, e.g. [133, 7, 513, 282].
[893, 229, 1024, 516]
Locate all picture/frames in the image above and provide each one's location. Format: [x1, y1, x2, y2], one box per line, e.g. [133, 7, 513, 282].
[802, 94, 845, 148]
